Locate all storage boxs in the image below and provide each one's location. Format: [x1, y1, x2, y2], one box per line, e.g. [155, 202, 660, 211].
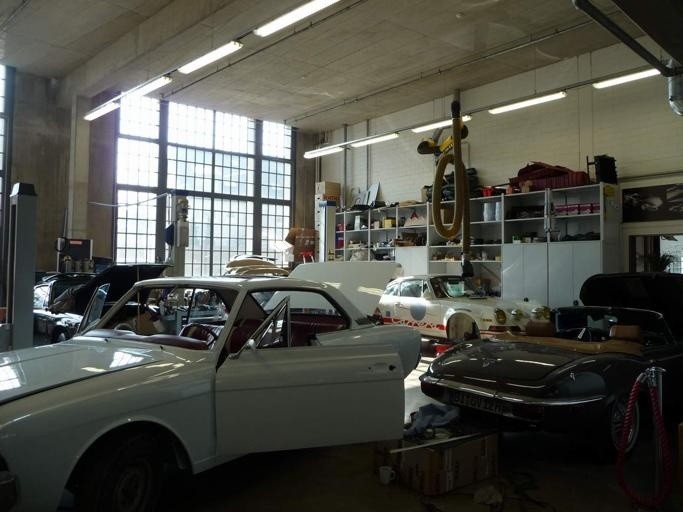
[373, 425, 493, 497]
[315, 221, 322, 231]
[314, 207, 323, 221]
[315, 181, 341, 195]
[315, 195, 340, 207]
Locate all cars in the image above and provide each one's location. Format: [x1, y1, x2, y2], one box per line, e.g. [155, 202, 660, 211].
[222, 255, 292, 278]
[33, 265, 173, 343]
[421, 272, 682, 456]
[378, 276, 552, 346]
[0, 261, 421, 512]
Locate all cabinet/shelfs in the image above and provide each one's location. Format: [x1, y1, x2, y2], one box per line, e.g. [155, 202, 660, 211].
[428, 199, 463, 275]
[463, 195, 503, 296]
[502, 188, 548, 243]
[548, 183, 621, 243]
[369, 205, 397, 262]
[501, 244, 548, 310]
[335, 211, 344, 261]
[548, 242, 602, 310]
[344, 208, 370, 262]
[397, 203, 428, 245]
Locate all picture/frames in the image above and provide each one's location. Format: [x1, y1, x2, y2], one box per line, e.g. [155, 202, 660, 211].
[617, 171, 683, 236]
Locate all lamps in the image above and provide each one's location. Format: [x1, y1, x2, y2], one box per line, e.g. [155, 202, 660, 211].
[350, 133, 399, 149]
[253, 0, 340, 38]
[177, 40, 244, 75]
[130, 76, 173, 101]
[84, 100, 121, 121]
[488, 90, 568, 115]
[303, 146, 345, 159]
[411, 115, 472, 133]
[592, 68, 662, 89]
